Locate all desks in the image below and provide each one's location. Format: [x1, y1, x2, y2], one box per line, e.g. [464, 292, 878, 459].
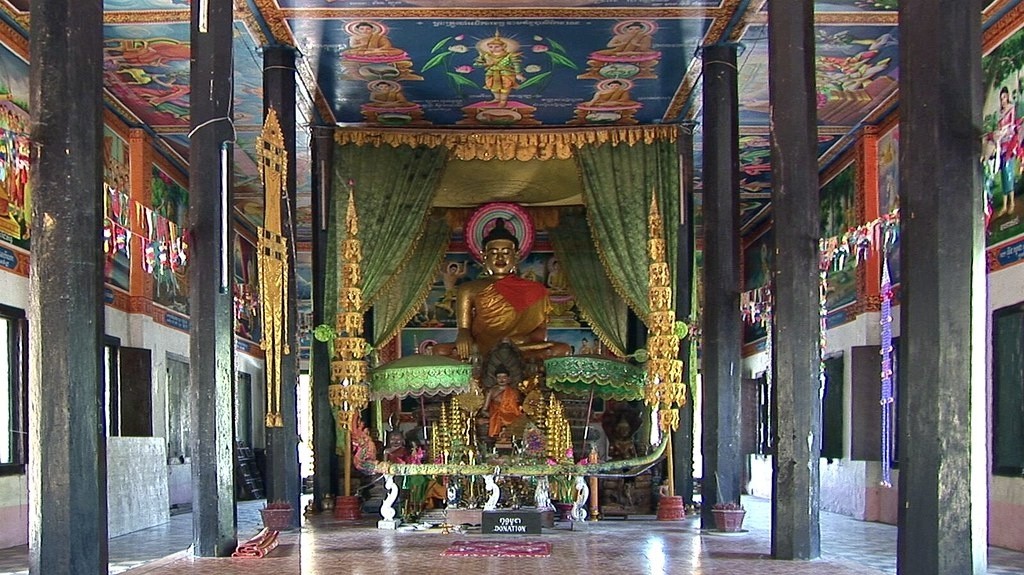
[481, 510, 541, 535]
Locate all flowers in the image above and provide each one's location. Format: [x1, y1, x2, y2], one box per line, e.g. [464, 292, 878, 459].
[545, 448, 588, 499]
[400, 448, 443, 503]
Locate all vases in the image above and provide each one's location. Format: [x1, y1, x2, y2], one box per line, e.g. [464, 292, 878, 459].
[556, 501, 573, 511]
[409, 502, 429, 514]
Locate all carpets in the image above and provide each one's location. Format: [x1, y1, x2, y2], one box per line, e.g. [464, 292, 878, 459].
[440, 540, 553, 558]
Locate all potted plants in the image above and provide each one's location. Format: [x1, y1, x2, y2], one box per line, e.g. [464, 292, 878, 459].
[711, 503, 746, 531]
[259, 497, 293, 531]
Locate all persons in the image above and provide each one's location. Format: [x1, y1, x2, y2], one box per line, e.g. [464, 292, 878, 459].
[382, 430, 409, 463]
[432, 217, 571, 363]
[481, 364, 521, 437]
[609, 414, 637, 461]
[449, 346, 462, 360]
[660, 476, 669, 497]
[497, 425, 511, 438]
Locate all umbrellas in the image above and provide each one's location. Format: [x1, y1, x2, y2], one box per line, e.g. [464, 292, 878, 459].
[544, 349, 646, 457]
[369, 335, 474, 439]
